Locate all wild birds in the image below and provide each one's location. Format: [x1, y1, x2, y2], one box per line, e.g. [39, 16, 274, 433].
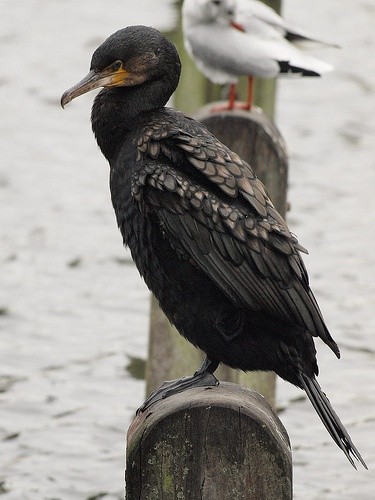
[180, 0, 341, 114]
[59, 24, 370, 472]
